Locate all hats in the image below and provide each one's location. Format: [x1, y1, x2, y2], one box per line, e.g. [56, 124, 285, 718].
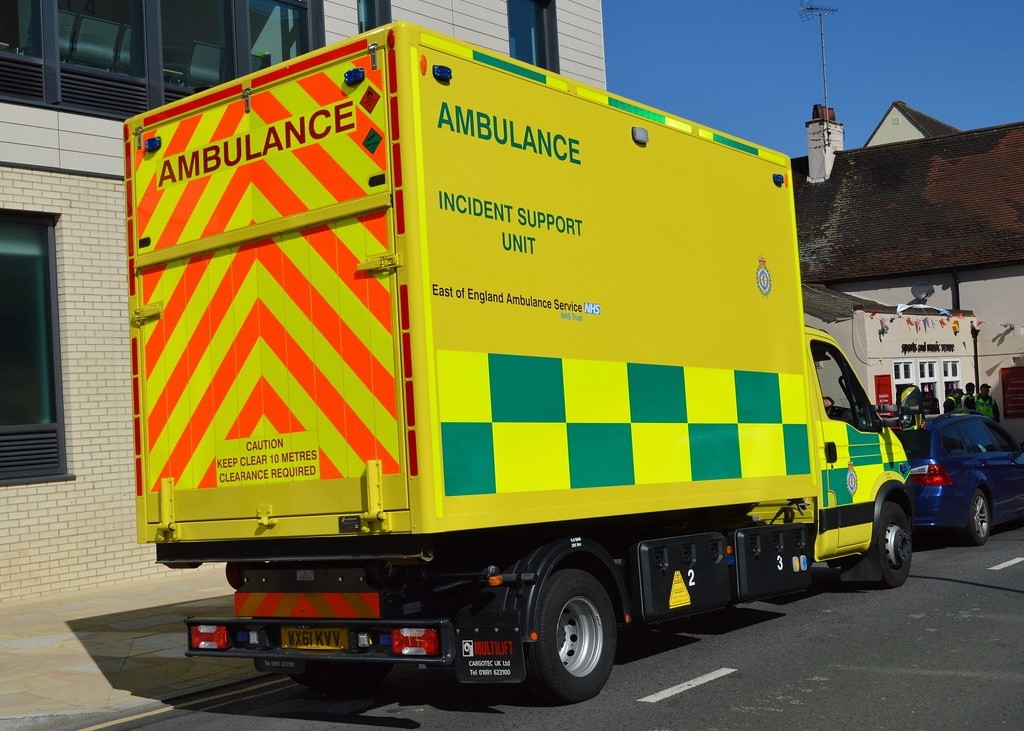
[981, 383, 991, 387]
[954, 388, 964, 395]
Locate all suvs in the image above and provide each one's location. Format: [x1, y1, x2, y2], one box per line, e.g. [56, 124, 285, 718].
[888, 410, 1024, 546]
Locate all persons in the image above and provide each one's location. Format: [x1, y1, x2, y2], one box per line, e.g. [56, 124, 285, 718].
[960, 382, 977, 414]
[975, 384, 1000, 423]
[943, 388, 964, 413]
[824, 397, 835, 408]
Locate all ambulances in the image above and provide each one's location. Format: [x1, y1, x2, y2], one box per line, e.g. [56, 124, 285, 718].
[122, 21, 926, 705]
